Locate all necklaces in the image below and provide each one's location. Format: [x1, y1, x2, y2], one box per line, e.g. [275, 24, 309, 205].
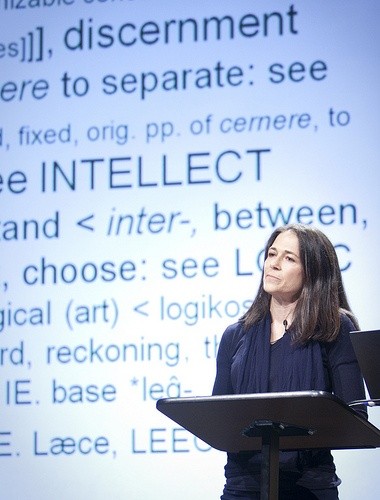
[268, 298, 301, 330]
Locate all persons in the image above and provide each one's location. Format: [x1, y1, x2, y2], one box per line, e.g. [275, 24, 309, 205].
[211, 226, 368, 500]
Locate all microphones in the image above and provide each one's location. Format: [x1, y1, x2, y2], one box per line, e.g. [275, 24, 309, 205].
[283, 320, 288, 332]
